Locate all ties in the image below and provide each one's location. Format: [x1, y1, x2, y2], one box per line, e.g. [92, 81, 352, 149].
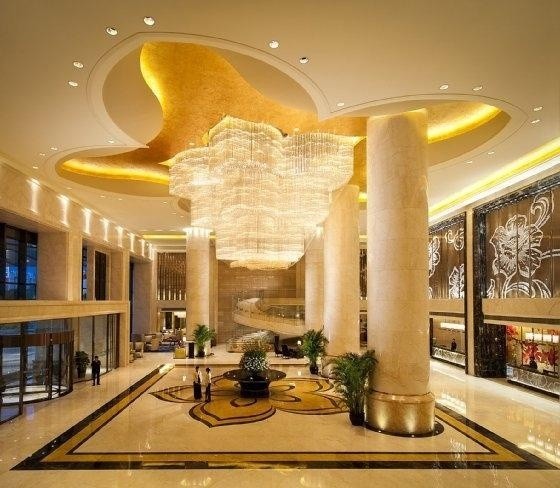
[198, 375, 200, 384]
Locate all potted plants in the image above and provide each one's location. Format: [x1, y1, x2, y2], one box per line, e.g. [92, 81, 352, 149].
[330, 349, 380, 426]
[73, 350, 91, 379]
[191, 323, 217, 357]
[296, 324, 330, 374]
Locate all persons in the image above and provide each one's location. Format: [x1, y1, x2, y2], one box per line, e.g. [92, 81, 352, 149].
[91, 355, 101, 386]
[204, 368, 212, 402]
[192, 366, 203, 401]
[529, 355, 537, 369]
[451, 338, 457, 350]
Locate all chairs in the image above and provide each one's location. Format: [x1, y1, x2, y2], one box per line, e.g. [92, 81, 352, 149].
[129, 328, 186, 363]
[273, 342, 296, 359]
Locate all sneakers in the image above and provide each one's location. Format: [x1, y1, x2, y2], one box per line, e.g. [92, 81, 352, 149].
[97, 384, 100, 385]
[208, 400, 211, 402]
[92, 385, 95, 386]
[205, 400, 208, 402]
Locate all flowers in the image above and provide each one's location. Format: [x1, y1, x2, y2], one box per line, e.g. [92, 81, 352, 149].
[239, 349, 272, 378]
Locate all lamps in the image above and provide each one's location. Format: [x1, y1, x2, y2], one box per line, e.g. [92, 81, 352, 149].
[158, 118, 368, 272]
